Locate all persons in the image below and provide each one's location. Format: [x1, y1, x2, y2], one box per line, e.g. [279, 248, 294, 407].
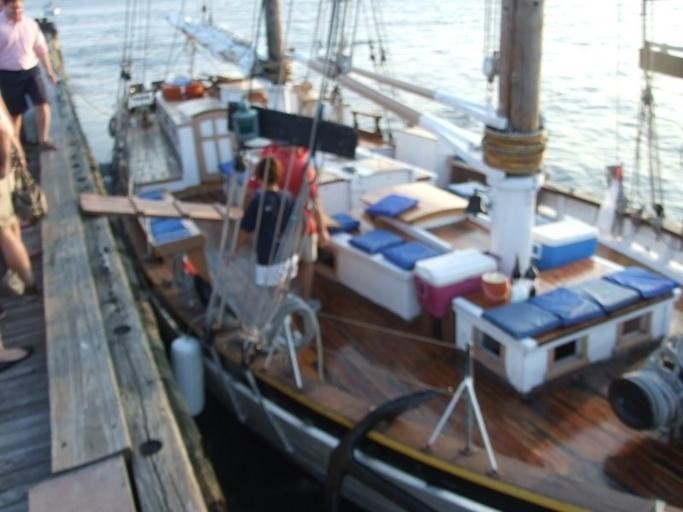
[243, 141, 330, 317]
[225, 157, 310, 342]
[0, 0, 58, 372]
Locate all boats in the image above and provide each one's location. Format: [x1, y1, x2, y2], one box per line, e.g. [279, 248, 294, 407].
[110, 0, 683, 512]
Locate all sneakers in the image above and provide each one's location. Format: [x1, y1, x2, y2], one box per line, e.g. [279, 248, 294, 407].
[276, 332, 302, 349]
[300, 299, 320, 318]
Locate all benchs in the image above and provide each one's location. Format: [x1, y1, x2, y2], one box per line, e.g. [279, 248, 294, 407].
[450, 254, 682, 394]
[315, 213, 445, 321]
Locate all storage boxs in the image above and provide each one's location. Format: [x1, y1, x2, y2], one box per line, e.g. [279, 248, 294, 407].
[533, 218, 598, 271]
[413, 247, 497, 320]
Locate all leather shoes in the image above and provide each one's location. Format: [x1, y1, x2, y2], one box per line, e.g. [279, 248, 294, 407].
[0, 342, 31, 371]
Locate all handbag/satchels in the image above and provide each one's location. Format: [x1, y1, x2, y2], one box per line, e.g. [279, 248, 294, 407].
[11, 154, 50, 222]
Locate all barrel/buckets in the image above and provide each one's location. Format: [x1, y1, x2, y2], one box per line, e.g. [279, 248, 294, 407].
[482, 274, 506, 307]
